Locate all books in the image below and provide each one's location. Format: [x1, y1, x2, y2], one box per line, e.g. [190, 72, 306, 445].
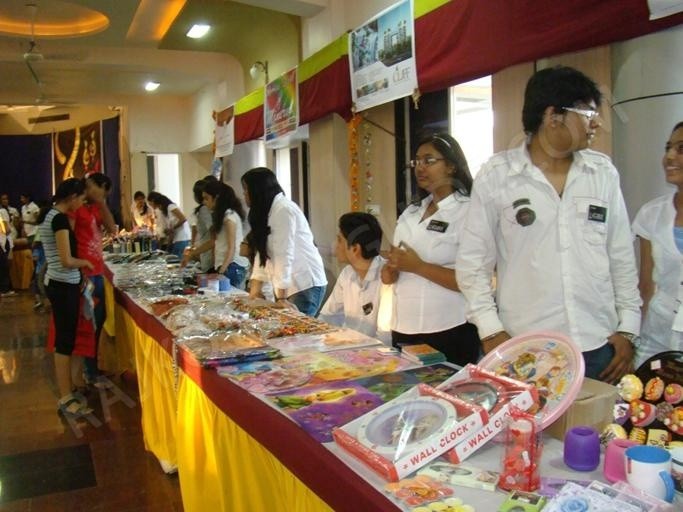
[401, 344, 447, 364]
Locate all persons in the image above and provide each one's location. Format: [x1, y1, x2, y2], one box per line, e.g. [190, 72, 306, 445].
[455, 66, 644, 386]
[241, 168, 327, 317]
[318, 211, 396, 346]
[633, 121, 683, 368]
[2, 172, 249, 415]
[377, 132, 477, 366]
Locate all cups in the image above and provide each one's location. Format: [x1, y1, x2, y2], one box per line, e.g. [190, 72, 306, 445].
[623, 444, 675, 504]
[565, 427, 632, 480]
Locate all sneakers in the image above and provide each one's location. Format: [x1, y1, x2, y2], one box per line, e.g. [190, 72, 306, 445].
[33, 301, 44, 310]
[1, 290, 15, 297]
[83, 372, 112, 389]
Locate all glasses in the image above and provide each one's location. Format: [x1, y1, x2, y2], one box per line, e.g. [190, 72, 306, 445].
[409, 157, 447, 170]
[556, 105, 599, 124]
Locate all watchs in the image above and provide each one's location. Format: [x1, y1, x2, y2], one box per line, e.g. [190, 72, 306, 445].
[622, 333, 641, 348]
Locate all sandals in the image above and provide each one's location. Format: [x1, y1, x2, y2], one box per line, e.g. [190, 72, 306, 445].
[57, 384, 91, 416]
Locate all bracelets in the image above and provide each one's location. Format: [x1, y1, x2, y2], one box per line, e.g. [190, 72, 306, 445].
[276, 298, 289, 300]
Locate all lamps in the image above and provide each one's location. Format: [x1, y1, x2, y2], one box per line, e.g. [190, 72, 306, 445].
[250, 59, 268, 84]
[17, 3, 49, 104]
